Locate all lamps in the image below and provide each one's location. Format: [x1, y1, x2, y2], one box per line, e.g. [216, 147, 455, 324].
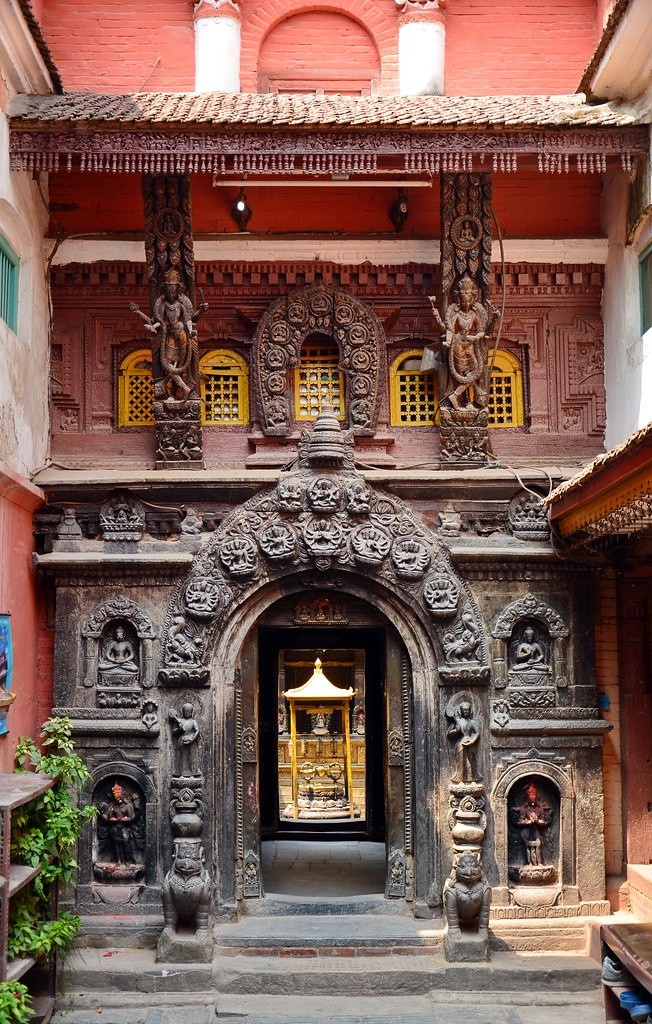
[213, 174, 433, 187]
[232, 188, 251, 232]
[389, 197, 408, 231]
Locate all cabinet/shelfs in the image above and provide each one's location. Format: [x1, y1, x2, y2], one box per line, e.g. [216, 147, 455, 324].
[600, 923, 652, 1024]
[0, 770, 57, 1024]
